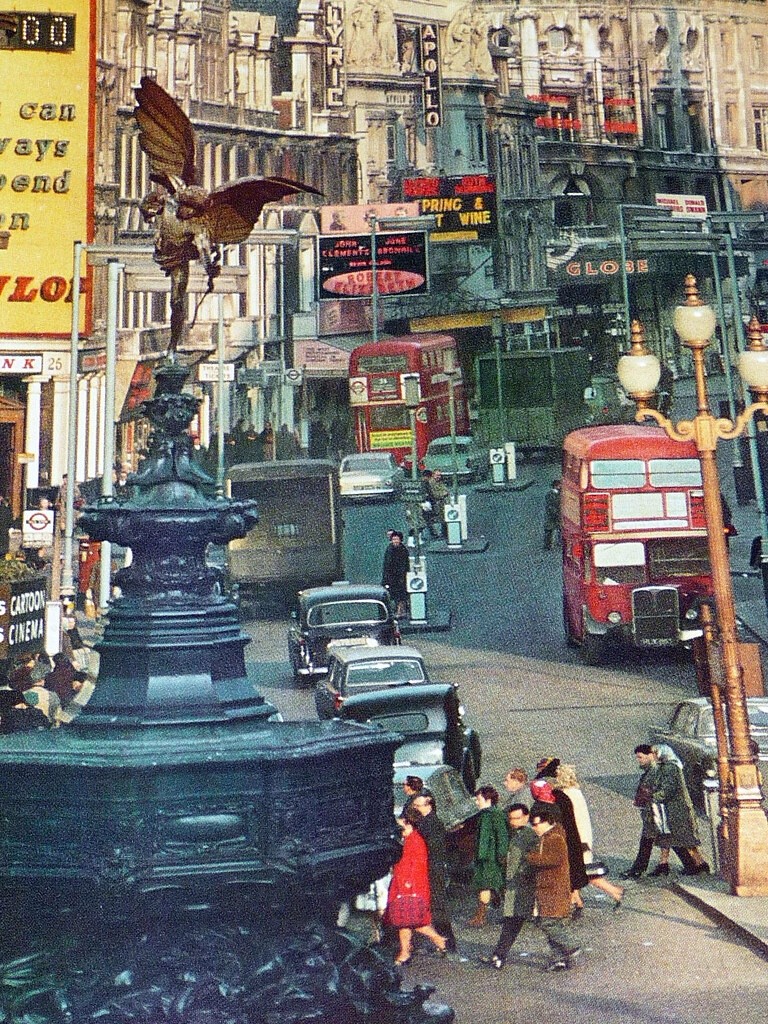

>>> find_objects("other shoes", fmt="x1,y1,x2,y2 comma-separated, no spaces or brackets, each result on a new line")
681,862,709,876
572,899,585,921
569,946,582,961
436,939,456,960
478,953,505,970
621,870,641,882
394,955,413,967
613,888,626,911
546,960,567,973
647,864,670,877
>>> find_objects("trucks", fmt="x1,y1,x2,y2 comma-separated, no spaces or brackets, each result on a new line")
473,347,620,462
224,459,345,604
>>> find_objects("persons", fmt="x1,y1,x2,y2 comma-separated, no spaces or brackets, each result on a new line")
113,462,132,495
39,499,50,509
382,530,411,617
0,489,14,551
618,745,711,878
0,615,85,731
465,758,626,970
57,474,81,532
720,493,732,550
141,184,220,351
75,548,122,620
408,469,449,549
0,549,51,580
192,416,346,477
336,776,457,966
544,480,562,549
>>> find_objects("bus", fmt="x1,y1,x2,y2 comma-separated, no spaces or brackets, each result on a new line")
349,334,472,474
561,426,729,668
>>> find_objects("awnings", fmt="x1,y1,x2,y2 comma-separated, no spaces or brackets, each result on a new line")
293,332,394,378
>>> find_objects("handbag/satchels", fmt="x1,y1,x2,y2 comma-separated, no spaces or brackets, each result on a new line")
587,862,608,878
390,887,427,928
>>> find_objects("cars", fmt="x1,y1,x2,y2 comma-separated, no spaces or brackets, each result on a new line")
418,435,482,485
314,646,431,722
285,581,401,689
389,763,478,834
646,694,766,808
340,451,403,505
342,683,482,794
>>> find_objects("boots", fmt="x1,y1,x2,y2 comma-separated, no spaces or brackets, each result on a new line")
469,900,489,926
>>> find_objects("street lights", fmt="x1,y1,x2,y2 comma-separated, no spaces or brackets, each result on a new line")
617,275,767,898
